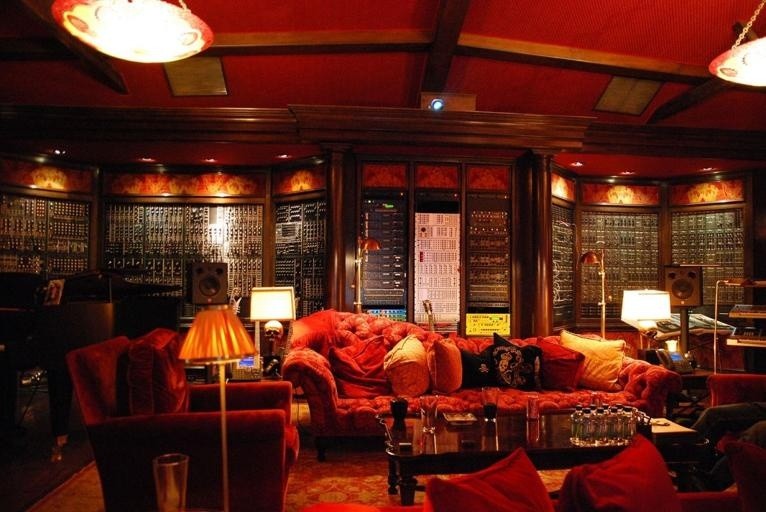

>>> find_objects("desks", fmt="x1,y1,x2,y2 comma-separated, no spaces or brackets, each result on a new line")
374,413,696,506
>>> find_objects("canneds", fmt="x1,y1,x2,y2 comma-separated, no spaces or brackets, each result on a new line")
527,397,539,419
527,419,539,442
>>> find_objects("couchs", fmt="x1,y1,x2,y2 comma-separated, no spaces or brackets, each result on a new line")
285,311,681,461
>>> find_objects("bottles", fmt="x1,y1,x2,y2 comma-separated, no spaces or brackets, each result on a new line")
570,404,636,447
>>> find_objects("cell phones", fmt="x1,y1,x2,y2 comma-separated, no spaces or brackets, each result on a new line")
460,438,474,446
398,440,413,449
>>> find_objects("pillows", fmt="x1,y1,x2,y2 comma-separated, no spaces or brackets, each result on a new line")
559,329,626,391
421,446,556,512
383,334,429,397
490,332,542,392
128,328,190,414
556,431,683,512
425,338,462,395
329,334,390,400
461,345,493,388
534,336,585,393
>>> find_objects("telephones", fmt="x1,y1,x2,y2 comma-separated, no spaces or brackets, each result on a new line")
655,348,694,374
226,357,264,382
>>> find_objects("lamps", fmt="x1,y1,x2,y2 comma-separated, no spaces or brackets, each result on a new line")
581,241,605,339
620,290,671,350
709,1,766,86
177,309,258,512
354,236,380,313
249,287,297,356
49,0,214,63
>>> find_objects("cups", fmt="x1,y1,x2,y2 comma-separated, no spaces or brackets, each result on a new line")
152,453,190,512
419,396,438,433
527,394,539,419
482,387,500,421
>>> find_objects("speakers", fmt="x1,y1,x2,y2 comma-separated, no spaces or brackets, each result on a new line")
663,264,703,309
186,262,228,306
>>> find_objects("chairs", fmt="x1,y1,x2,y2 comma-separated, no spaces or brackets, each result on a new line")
708,374,766,452
67,336,300,512
